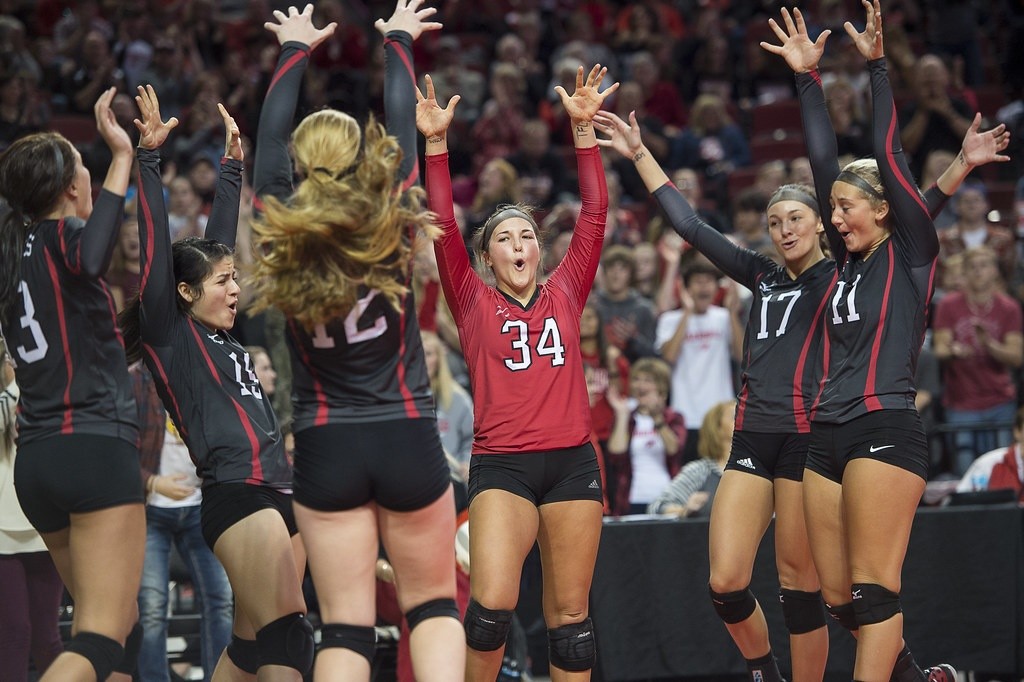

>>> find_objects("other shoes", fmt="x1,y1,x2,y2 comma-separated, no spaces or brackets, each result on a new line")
923,664,958,682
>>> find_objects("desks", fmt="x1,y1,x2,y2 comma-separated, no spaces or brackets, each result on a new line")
515,504,1024,682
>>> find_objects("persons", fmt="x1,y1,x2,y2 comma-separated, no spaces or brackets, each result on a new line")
759,0,963,682
240,0,467,682
414,65,619,682
0,87,146,682
0,0,1024,682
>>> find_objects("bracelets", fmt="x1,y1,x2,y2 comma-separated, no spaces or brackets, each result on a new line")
150,475,157,493
